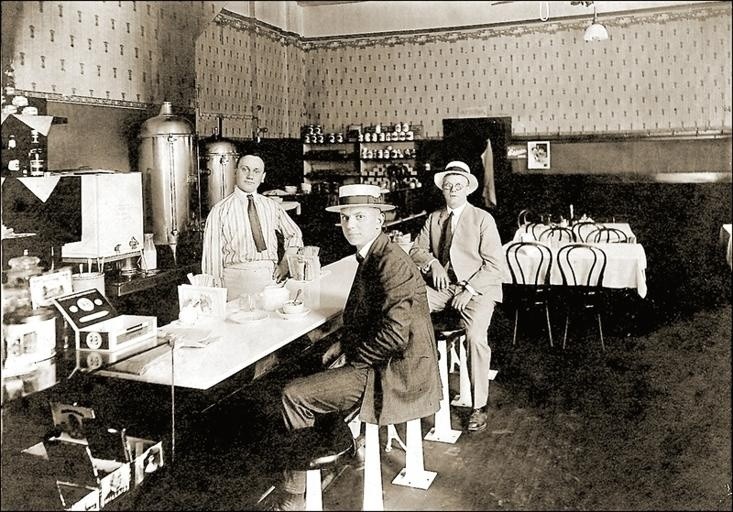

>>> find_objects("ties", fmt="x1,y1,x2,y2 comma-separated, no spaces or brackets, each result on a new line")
245,195,269,252
438,211,455,267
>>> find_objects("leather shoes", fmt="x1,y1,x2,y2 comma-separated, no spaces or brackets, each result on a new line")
310,438,357,468
468,407,488,430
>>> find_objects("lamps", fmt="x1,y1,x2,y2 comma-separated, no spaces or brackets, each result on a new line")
582,1,611,42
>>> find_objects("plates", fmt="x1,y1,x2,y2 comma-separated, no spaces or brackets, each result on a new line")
275,307,312,319
226,310,272,325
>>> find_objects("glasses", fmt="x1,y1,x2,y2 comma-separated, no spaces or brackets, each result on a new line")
442,183,465,192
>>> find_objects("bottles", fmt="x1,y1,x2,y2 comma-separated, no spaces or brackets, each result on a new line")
142,232,158,273
292,256,314,281
27,129,46,178
5,134,23,177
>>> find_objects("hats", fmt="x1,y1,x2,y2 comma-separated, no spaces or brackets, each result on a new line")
324,184,396,212
434,161,479,196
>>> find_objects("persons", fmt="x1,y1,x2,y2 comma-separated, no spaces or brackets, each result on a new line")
201,153,303,301
408,160,506,433
266,185,443,512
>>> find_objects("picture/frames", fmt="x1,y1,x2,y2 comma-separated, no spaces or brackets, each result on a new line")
525,139,552,172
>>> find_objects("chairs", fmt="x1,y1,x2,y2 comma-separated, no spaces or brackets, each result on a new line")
500,208,646,354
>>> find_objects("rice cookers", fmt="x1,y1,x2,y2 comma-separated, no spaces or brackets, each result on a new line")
1,302,60,370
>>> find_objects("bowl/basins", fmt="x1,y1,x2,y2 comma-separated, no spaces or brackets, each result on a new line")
284,186,298,195
300,182,312,193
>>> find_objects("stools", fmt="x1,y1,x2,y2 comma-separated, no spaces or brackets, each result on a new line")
423,317,470,448
262,425,355,512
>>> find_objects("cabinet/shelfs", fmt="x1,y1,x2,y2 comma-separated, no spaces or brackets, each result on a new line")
299,122,422,193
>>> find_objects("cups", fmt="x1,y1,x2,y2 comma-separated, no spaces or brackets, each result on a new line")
238,293,266,312
282,300,305,314
